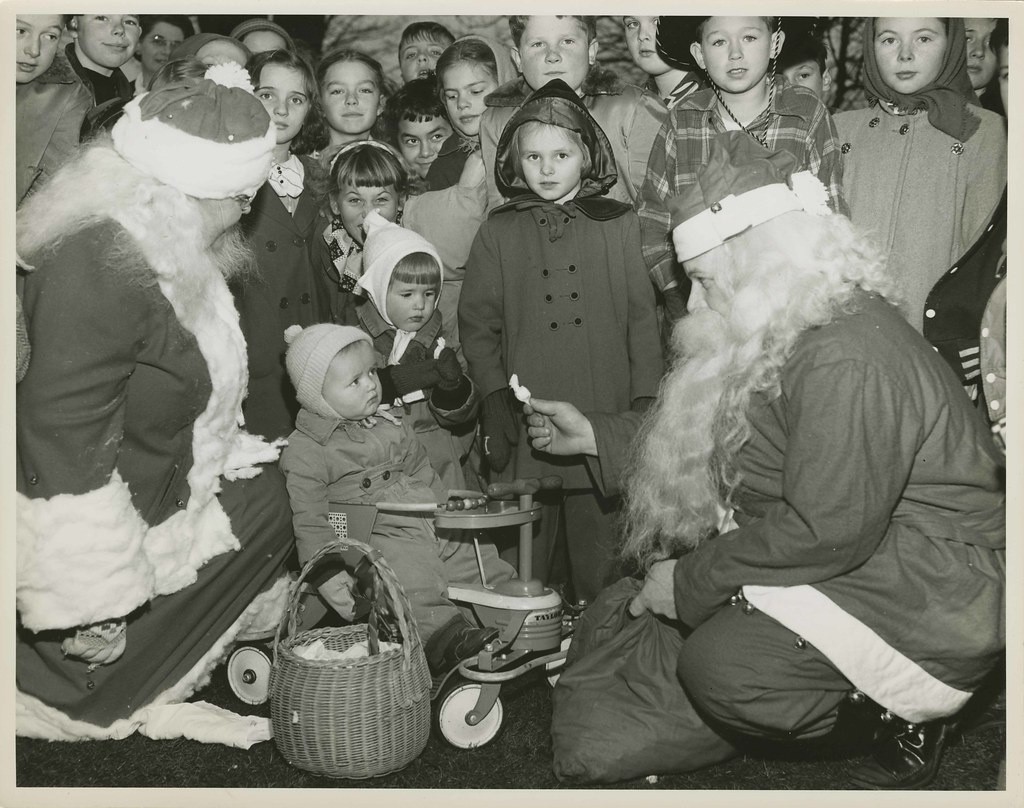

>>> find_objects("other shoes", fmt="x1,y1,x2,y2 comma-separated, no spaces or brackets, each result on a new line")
453,625,503,665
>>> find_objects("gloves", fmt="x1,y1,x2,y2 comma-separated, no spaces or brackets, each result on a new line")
481,391,524,470
389,359,442,396
60,616,130,666
434,346,470,394
321,566,364,625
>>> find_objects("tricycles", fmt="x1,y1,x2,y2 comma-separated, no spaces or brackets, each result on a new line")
225,474,584,751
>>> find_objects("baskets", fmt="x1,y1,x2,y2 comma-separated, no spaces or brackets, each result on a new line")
278,537,433,781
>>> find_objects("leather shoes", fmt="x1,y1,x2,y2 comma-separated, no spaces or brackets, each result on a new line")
852,708,961,790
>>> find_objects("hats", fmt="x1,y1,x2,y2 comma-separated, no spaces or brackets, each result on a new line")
667,129,831,266
112,77,284,199
283,322,378,422
654,12,814,71
359,211,444,328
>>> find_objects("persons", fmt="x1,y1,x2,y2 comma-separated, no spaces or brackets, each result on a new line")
459,80,662,600
524,132,1006,791
16,15,1010,450
284,323,518,679
351,210,480,492
16,62,303,740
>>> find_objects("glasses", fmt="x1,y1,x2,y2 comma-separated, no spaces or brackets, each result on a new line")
150,34,186,55
236,190,257,211
